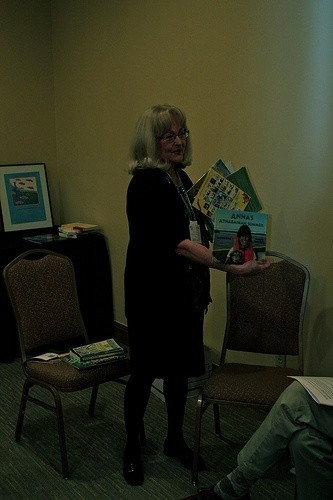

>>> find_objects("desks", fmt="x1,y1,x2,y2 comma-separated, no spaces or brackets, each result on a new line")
0,226,113,365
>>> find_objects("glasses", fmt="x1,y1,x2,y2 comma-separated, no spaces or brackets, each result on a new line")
156,129,189,144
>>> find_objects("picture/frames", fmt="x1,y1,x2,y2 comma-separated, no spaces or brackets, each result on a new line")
0,163,55,237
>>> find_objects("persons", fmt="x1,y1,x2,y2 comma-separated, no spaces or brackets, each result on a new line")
123,105,271,487
287,376,333,406
226,225,257,264
179,379,333,500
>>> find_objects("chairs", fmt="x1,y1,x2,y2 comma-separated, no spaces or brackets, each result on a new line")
192,251,311,487
3,249,132,481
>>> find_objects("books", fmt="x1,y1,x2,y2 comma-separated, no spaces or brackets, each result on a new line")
60,338,128,371
186,159,265,243
23,234,69,244
212,207,269,264
58,222,101,239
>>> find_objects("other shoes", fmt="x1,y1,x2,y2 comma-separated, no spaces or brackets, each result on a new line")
123,445,143,486
181,483,251,500
163,439,207,471
